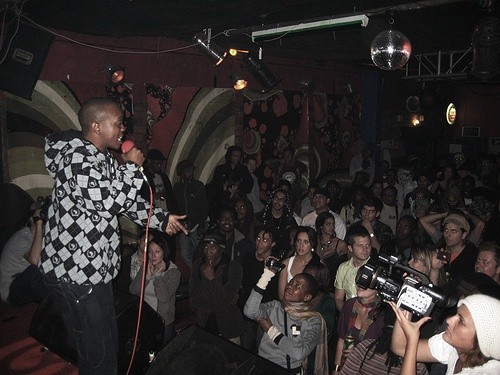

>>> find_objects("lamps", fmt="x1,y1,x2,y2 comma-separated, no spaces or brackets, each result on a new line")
370,12,412,70
244,55,283,94
229,71,248,91
252,15,369,41
445,102,457,125
106,65,127,85
195,35,227,67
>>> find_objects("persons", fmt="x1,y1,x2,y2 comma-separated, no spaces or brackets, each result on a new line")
239,227,285,354
190,230,243,348
0,217,58,309
37,98,191,375
243,255,329,375
426,273,500,374
278,226,319,302
328,286,393,374
383,294,500,375
171,160,209,289
117,235,183,374
303,261,336,374
205,145,500,286
113,230,155,296
333,224,372,311
342,307,430,375
139,149,178,263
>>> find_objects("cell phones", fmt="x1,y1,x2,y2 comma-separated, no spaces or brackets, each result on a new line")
437,251,451,264
266,258,286,274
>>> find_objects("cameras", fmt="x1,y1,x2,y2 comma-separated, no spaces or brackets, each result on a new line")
354,251,464,325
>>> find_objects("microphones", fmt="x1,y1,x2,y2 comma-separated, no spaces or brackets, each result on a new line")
121,140,144,173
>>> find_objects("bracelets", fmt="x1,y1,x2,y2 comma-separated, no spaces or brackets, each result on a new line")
332,363,341,371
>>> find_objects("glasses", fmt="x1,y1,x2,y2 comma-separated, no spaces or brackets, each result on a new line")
295,239,311,244
255,236,269,243
362,208,376,214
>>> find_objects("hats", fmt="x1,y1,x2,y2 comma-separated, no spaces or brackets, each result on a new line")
176,159,197,176
203,230,227,246
442,213,470,236
456,294,500,360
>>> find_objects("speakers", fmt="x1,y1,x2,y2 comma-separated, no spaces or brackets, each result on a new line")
0,18,56,102
